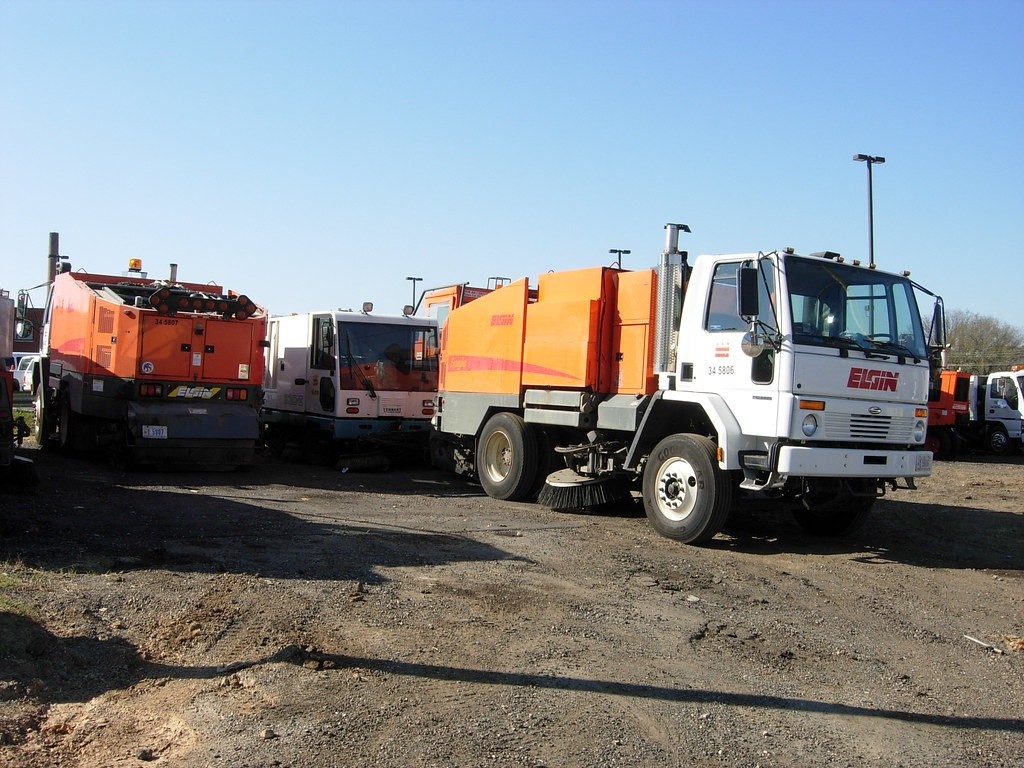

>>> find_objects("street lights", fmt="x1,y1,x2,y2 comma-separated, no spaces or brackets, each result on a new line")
406,276,422,311
854,153,887,337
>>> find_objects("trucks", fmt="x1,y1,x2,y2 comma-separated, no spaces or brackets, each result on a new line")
423,221,948,546
926,364,1024,455
261,302,439,447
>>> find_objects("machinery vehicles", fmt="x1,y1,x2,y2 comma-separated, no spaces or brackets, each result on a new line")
405,277,512,361
9,232,273,453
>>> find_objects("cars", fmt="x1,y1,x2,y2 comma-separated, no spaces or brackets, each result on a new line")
12,352,41,393
